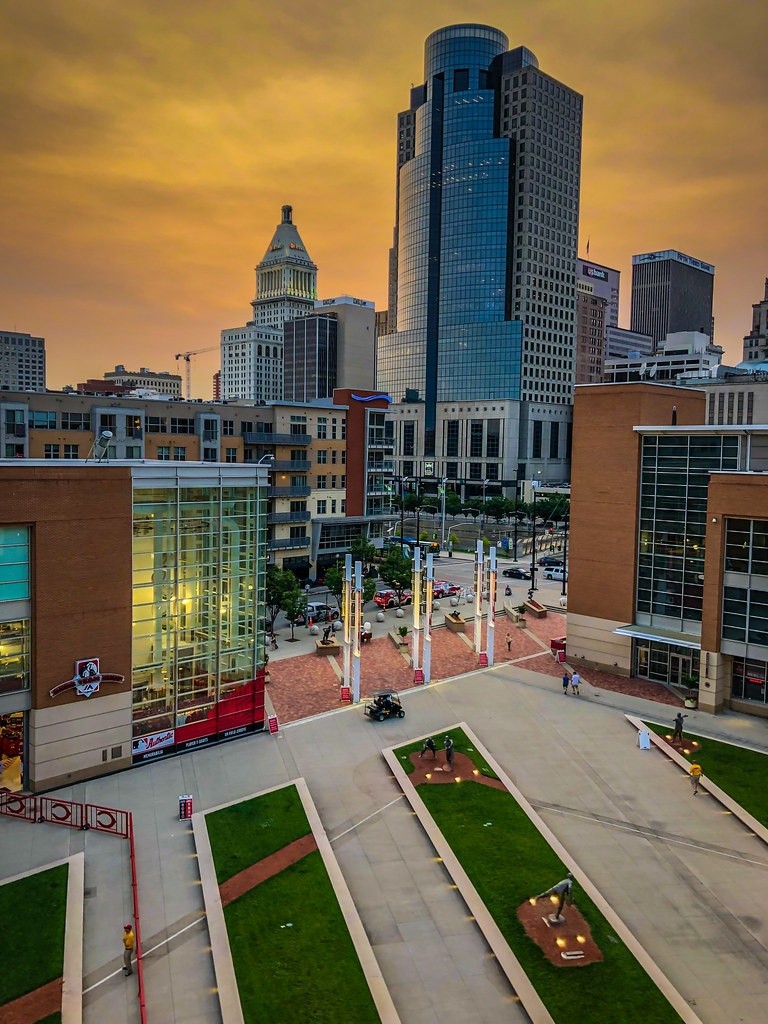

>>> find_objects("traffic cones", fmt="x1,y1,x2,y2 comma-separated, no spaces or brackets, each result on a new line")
308,616,313,626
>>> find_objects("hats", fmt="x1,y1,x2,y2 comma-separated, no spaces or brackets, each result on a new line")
124,925,132,930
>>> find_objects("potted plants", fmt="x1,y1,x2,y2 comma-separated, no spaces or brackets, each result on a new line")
518,606,526,627
399,626,408,652
685,674,698,708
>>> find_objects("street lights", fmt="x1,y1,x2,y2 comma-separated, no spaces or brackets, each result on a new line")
304,584,310,628
400,475,409,555
441,477,449,551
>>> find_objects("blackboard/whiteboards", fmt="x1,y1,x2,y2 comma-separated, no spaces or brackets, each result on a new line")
636,732,650,750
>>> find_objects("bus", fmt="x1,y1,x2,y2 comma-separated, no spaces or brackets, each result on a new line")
407,541,441,562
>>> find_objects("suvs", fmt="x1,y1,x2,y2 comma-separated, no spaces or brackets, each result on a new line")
297,601,340,625
372,588,412,609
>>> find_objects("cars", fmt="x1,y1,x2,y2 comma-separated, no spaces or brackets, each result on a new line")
536,556,564,567
542,566,568,582
502,566,532,580
433,579,461,599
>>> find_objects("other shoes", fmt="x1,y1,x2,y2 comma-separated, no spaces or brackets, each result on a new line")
573,693,575,694
693,791,698,795
564,692,567,694
577,691,579,694
123,967,128,970
125,972,132,976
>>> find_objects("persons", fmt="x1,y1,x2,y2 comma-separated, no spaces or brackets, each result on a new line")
689,760,702,795
376,694,393,710
0,720,17,735
535,873,574,920
670,712,684,746
528,589,534,603
451,610,460,618
505,632,512,652
563,673,569,695
504,585,512,606
420,736,436,758
142,689,173,712
322,626,331,645
331,620,338,637
270,634,276,651
443,735,454,771
122,925,135,977
570,672,582,695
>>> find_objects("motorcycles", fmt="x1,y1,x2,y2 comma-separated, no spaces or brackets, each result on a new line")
363,689,406,722
